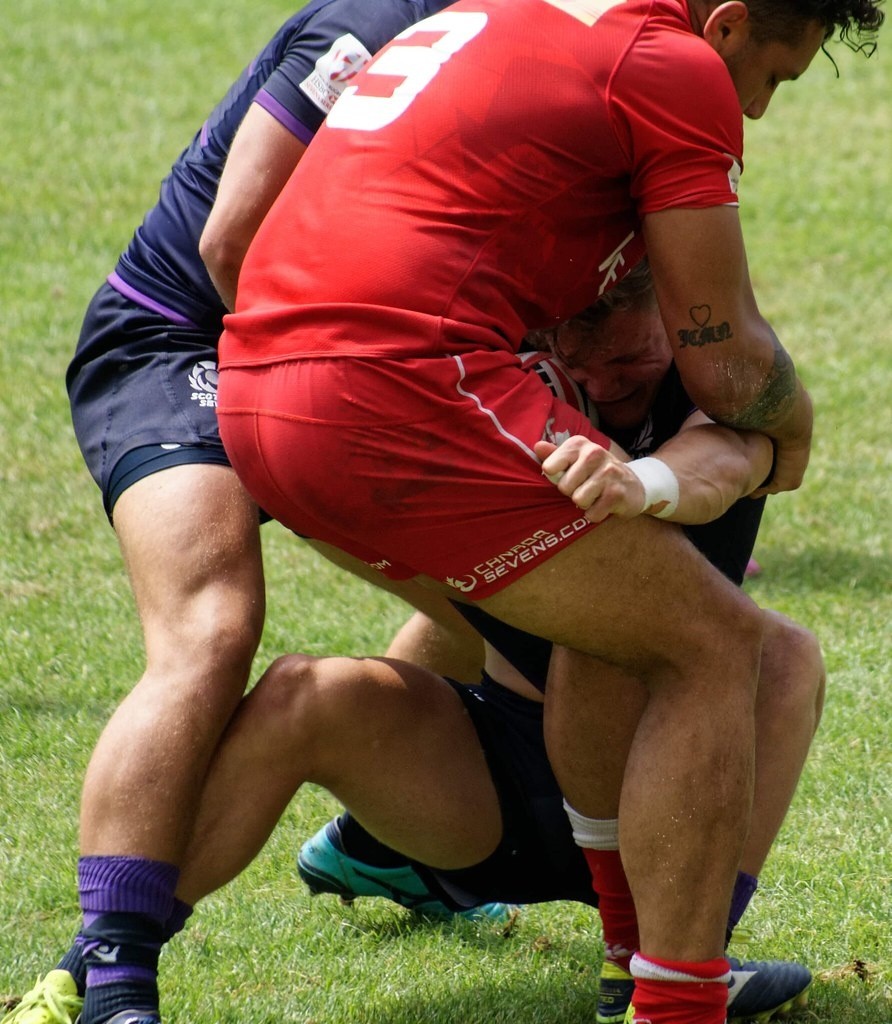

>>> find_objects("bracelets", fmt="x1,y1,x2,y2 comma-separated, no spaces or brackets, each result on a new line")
625,456,680,519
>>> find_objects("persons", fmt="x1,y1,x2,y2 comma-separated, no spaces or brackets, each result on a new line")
0,0,884,1024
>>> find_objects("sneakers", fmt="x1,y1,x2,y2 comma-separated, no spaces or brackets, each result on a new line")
595,953,814,1024
0,969,84,1024
297,816,510,934
